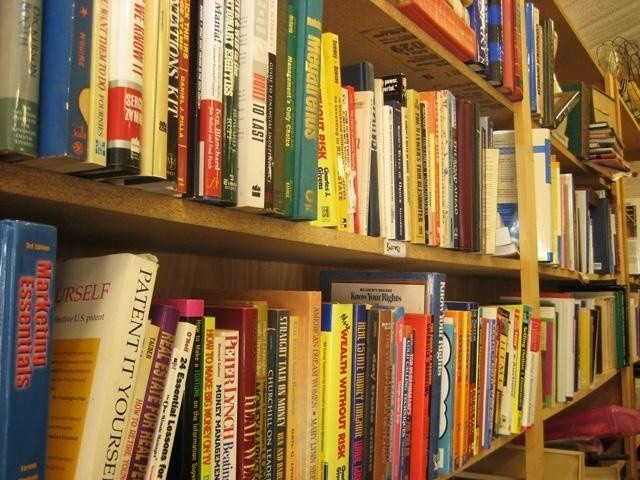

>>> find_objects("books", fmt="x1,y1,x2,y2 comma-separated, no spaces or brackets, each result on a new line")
2,0,639,479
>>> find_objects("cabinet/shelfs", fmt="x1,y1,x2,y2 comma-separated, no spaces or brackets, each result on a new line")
0,0,640,479
